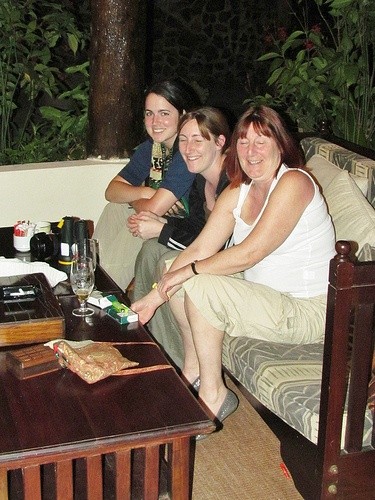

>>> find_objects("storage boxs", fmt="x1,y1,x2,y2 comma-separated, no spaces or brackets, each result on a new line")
6,344,62,380
0,273,66,347
86,291,139,324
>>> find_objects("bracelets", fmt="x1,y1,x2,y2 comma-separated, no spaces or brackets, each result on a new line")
151,282,158,290
191,259,200,275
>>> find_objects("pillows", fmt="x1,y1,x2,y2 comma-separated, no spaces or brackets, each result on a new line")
306,153,375,256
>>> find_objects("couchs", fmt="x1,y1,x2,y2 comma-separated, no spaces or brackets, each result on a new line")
222,137,375,500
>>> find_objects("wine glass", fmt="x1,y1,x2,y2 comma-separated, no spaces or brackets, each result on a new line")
70,257,96,317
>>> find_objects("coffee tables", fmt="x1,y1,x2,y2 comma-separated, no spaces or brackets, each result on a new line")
0,222,224,500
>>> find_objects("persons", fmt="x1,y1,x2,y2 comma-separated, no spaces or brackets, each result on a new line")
130,106,246,374
128,103,339,441
88,76,203,294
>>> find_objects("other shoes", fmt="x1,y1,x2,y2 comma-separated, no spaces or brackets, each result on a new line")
190,387,238,440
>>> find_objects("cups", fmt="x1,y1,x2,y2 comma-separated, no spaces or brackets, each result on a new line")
13,235,32,252
73,239,97,272
34,222,51,234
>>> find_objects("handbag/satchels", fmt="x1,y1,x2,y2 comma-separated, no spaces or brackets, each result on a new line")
53,341,140,385
